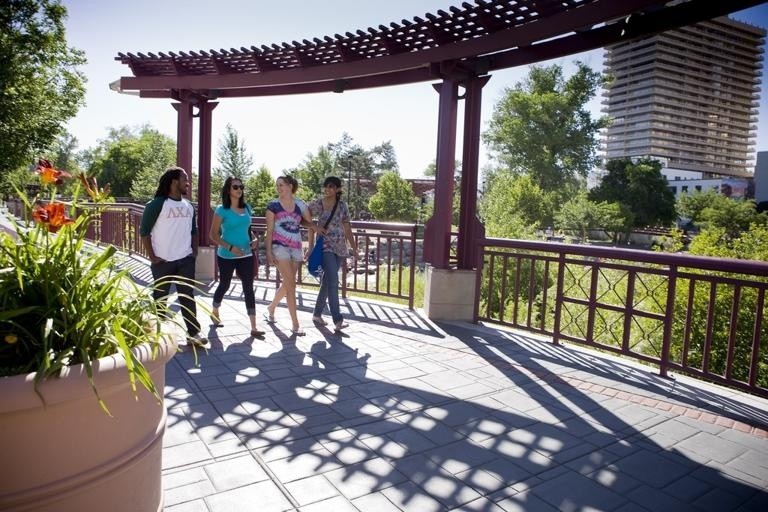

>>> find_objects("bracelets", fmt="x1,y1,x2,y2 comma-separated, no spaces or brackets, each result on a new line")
229,245,233,251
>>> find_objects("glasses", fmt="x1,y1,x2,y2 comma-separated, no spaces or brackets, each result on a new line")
231,185,245,190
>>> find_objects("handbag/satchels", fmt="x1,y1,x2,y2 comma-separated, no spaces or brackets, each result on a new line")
307,236,324,278
252,252,259,278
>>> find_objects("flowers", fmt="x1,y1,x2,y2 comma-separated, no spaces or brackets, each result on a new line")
0,154,222,416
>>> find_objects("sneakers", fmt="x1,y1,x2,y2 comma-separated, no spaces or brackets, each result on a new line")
311,315,328,326
333,321,350,331
186,336,208,347
210,313,224,327
250,328,266,336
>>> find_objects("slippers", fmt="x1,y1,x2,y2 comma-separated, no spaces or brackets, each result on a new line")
263,311,277,323
290,326,306,336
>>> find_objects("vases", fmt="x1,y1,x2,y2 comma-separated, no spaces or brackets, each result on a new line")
0,310,180,511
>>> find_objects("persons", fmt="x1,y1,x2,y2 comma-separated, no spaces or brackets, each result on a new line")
301,177,357,331
266,175,315,335
209,176,265,336
140,168,208,347
